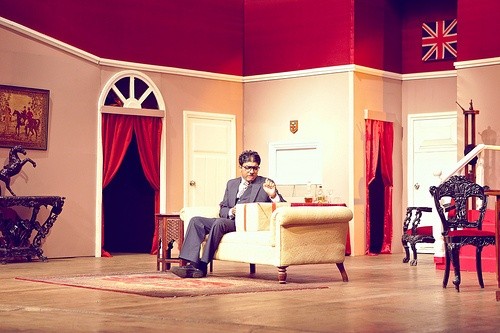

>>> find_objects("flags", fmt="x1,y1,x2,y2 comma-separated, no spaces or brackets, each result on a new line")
421,19,458,61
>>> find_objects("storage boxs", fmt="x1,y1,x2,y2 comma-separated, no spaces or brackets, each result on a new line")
234,202,292,233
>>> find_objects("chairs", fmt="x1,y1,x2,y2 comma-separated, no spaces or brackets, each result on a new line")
429,175,496,291
402,203,450,267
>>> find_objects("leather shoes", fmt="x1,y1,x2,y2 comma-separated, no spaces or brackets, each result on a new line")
170,265,206,278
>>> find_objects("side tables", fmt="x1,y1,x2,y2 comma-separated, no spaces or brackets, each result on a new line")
155,214,186,271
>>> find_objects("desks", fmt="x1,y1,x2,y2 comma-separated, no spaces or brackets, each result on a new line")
0,195,66,264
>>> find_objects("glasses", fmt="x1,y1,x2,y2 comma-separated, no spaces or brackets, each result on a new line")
242,165,261,170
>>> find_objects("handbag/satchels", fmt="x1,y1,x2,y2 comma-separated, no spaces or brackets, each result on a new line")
234,201,272,231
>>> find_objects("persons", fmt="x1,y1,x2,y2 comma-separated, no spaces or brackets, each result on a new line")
170,150,287,278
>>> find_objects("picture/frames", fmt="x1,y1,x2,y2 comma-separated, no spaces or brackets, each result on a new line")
0,85,50,150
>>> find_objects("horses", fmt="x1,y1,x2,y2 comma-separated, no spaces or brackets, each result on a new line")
0,145,36,196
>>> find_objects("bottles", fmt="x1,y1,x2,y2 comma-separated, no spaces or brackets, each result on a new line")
317,186,325,203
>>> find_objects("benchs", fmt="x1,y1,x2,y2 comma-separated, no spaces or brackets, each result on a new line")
180,206,354,283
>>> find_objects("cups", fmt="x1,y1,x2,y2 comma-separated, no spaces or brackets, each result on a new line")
304,193,313,203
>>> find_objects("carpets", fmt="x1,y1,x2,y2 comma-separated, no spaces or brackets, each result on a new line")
14,274,329,297
